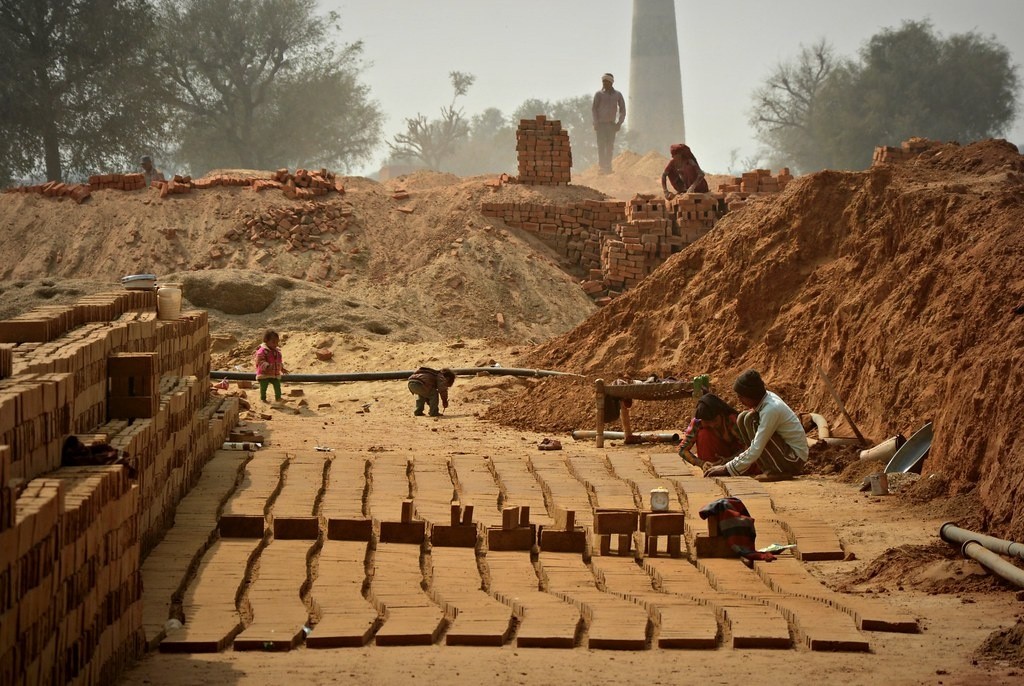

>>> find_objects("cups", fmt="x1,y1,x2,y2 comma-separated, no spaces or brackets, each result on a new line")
651,489,669,509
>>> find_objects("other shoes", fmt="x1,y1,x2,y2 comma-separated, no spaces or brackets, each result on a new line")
754,470,794,482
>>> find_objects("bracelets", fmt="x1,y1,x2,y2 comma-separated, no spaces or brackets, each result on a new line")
663,189,668,192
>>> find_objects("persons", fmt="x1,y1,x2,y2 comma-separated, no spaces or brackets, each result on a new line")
592,73,626,177
254,329,289,404
140,154,165,187
704,370,810,480
661,144,709,201
406,367,456,417
677,394,762,476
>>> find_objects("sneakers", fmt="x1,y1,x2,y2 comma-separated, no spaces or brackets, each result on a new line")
539,437,562,450
